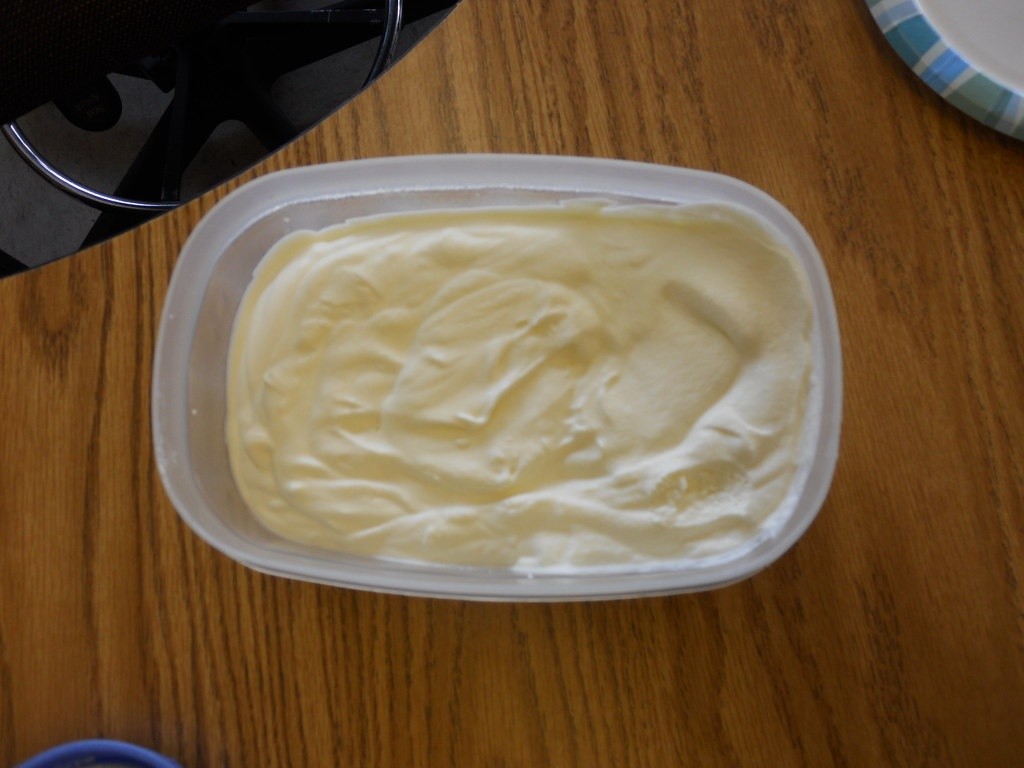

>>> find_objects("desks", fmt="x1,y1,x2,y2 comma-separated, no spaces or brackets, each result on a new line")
0,0,1024,768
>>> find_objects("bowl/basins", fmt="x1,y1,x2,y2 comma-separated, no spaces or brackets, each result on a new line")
14,738,181,768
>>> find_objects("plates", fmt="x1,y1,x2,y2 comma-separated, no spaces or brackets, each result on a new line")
865,0,1024,144
